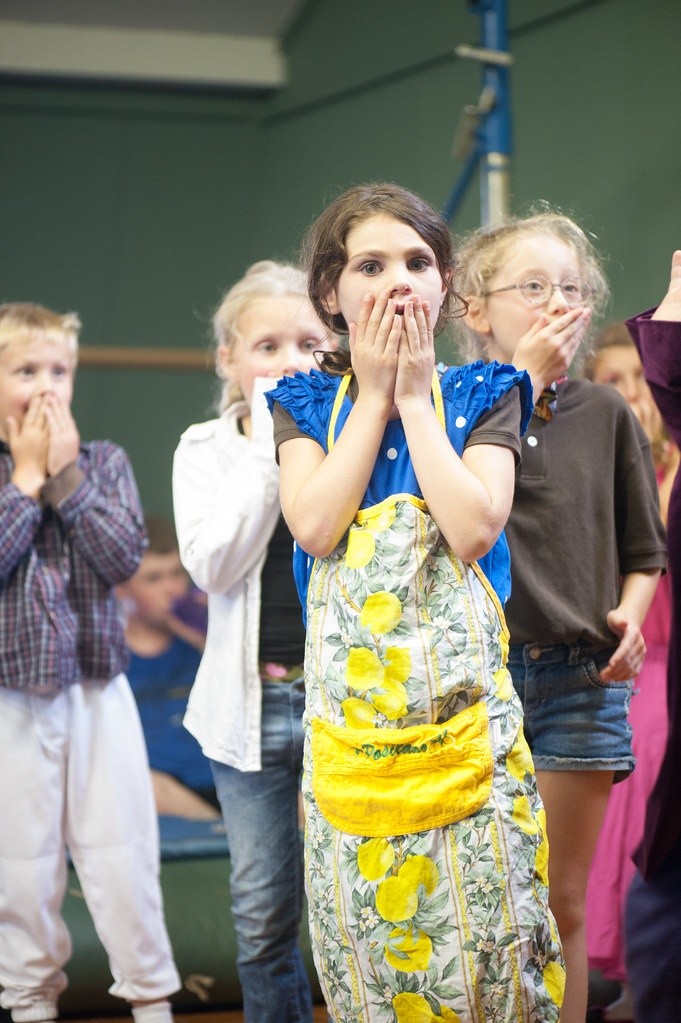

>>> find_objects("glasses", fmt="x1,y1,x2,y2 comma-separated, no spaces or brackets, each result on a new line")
483,272,596,308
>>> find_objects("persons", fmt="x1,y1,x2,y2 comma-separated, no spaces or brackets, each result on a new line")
0,297,180,1023
624,231,681,1022
453,209,668,1023
103,507,220,817
171,261,391,1023
573,325,679,1023
263,180,565,1022
